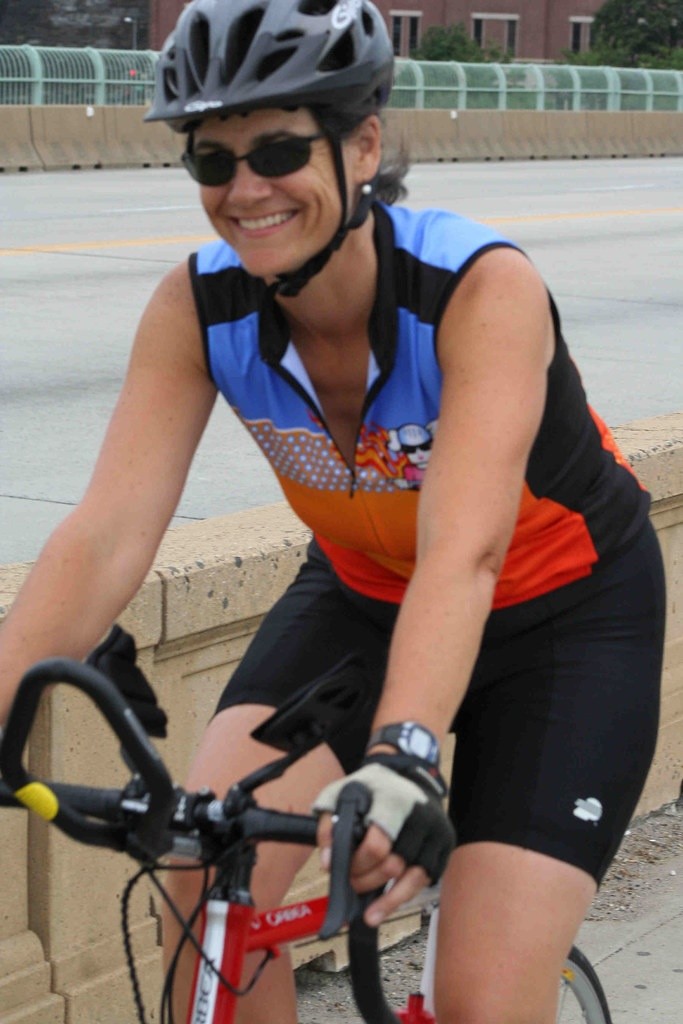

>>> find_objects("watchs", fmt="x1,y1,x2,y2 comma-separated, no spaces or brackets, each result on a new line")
367,720,440,766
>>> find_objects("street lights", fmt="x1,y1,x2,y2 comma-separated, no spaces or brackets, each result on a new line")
123,17,137,50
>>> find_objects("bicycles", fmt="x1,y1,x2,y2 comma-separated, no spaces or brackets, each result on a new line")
1,657,617,1023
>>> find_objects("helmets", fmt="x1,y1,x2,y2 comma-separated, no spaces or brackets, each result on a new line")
143,0,392,121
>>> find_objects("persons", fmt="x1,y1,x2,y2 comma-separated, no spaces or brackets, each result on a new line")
0,0,667,1024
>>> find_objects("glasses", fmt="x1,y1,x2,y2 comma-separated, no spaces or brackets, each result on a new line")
181,132,326,185
